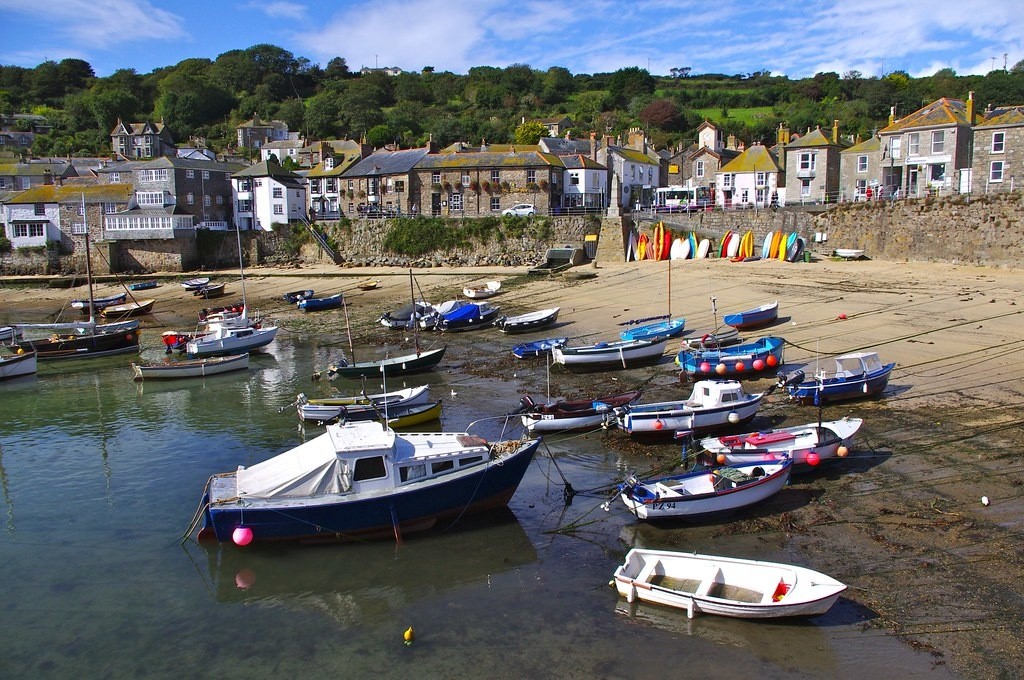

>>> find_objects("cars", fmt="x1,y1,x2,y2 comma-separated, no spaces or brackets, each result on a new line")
502,204,538,218
360,205,396,218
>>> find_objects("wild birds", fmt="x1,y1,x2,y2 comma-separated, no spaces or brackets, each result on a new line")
403,626,416,646
982,495,993,507
451,389,458,396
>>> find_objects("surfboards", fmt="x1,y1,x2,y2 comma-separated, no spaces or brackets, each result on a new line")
626,220,804,262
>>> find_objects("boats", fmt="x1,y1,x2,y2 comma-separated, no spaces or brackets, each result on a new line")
521,390,643,434
357,281,378,291
192,283,225,300
681,327,738,347
700,417,865,466
198,420,541,546
551,342,666,374
297,294,343,314
70,292,126,316
160,304,278,360
675,337,785,378
180,277,209,291
617,458,794,522
626,222,807,263
131,353,250,383
609,549,847,619
283,290,315,304
619,317,685,343
297,383,441,429
723,301,778,329
379,300,500,333
1,190,141,380
129,281,157,291
329,268,447,378
613,379,765,438
512,336,570,359
836,249,865,260
496,307,561,333
99,299,155,319
462,280,502,299
784,351,896,405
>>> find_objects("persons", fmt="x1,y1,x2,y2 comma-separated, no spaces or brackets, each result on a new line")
865,185,872,201
309,207,316,221
879,184,884,200
357,204,363,216
854,186,859,202
769,191,780,208
381,204,401,218
411,204,417,218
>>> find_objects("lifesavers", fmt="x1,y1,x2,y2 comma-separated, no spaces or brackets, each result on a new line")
701,334,716,352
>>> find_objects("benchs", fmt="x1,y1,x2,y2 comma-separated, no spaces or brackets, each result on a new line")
760,574,783,605
695,565,720,597
635,559,659,583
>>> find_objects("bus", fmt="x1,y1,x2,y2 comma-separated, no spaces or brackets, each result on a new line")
650,185,711,213
